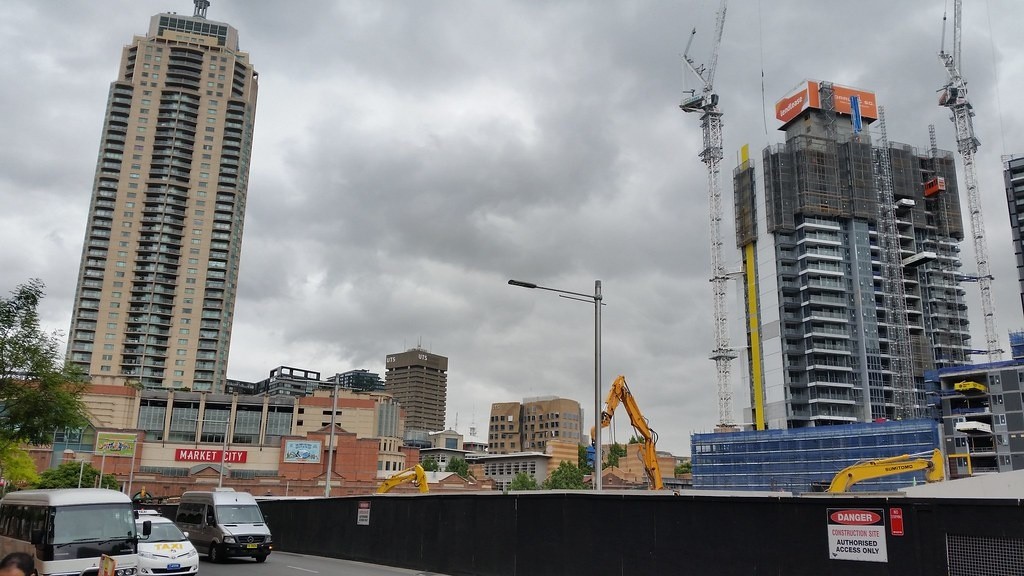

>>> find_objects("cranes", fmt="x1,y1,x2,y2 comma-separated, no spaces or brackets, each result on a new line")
935,1,1005,364
677,1,740,433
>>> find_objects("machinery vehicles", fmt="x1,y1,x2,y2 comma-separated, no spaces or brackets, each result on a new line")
585,375,665,490
827,448,946,492
377,463,430,494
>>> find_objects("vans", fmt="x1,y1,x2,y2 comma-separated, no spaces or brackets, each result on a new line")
174,489,274,563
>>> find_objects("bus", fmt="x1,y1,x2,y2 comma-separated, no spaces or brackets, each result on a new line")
1,487,135,575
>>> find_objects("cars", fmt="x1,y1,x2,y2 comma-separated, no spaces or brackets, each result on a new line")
134,508,199,575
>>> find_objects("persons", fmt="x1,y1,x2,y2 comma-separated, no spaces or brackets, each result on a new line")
0,551,37,576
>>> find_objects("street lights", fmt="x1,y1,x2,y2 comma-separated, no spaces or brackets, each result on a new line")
275,373,363,498
180,417,236,488
73,446,118,488
507,279,606,490
98,434,146,497
58,456,96,488
